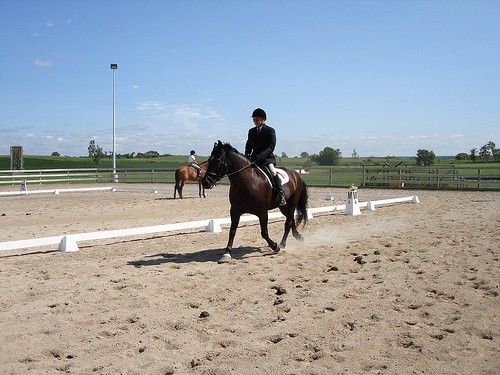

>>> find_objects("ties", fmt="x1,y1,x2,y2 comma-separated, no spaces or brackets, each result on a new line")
257,127,260,133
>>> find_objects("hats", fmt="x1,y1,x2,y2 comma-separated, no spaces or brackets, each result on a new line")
190,151,195,155
252,108,266,120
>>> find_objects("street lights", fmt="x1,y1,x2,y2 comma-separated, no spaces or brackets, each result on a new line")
111,63,118,181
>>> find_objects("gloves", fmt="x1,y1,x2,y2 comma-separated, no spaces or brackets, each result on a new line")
245,150,251,158
255,155,263,161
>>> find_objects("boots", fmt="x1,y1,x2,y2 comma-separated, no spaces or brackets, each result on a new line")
274,175,287,205
197,168,200,180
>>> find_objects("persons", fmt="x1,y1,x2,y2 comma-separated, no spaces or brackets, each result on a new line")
245,108,286,207
189,150,202,180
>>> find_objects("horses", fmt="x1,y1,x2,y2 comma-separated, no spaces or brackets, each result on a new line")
202,139,309,264
173,160,206,199
